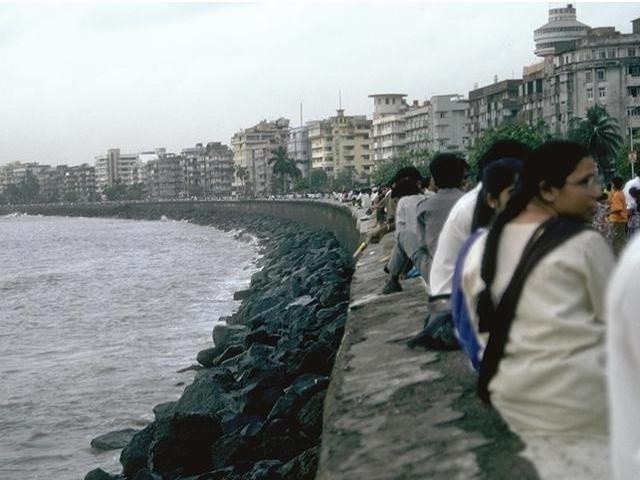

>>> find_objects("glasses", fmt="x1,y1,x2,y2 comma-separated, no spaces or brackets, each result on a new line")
564,175,604,191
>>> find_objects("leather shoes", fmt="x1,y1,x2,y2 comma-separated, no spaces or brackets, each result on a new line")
379,281,402,294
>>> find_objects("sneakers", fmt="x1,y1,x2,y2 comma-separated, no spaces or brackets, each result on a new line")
360,215,370,221
